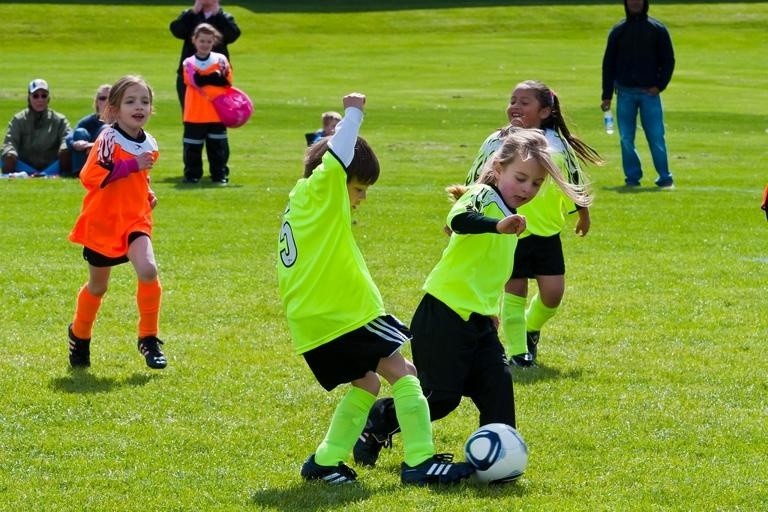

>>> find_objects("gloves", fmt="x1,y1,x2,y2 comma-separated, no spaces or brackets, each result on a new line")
3,153,16,172
61,149,72,171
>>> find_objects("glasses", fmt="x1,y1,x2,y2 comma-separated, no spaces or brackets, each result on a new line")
98,96,107,100
32,94,48,98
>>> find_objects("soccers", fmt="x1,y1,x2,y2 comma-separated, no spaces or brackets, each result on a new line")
465,423,527,483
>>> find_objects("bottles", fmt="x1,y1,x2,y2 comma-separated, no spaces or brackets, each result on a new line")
603,105,614,134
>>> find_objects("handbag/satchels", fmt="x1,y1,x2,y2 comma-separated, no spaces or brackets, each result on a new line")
212,87,253,127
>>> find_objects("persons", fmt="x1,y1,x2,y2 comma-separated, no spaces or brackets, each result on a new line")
178,23,235,187
64,80,113,177
312,107,341,139
66,73,173,372
2,73,73,181
596,3,676,188
342,112,596,476
466,76,609,367
167,1,243,127
277,91,475,486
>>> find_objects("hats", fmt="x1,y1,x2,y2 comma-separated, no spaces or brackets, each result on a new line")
29,79,49,93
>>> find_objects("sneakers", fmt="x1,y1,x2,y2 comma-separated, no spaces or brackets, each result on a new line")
354,398,400,466
510,353,539,370
69,324,90,367
138,335,166,369
302,454,357,486
182,177,200,183
213,177,228,186
527,331,540,360
401,454,475,484
